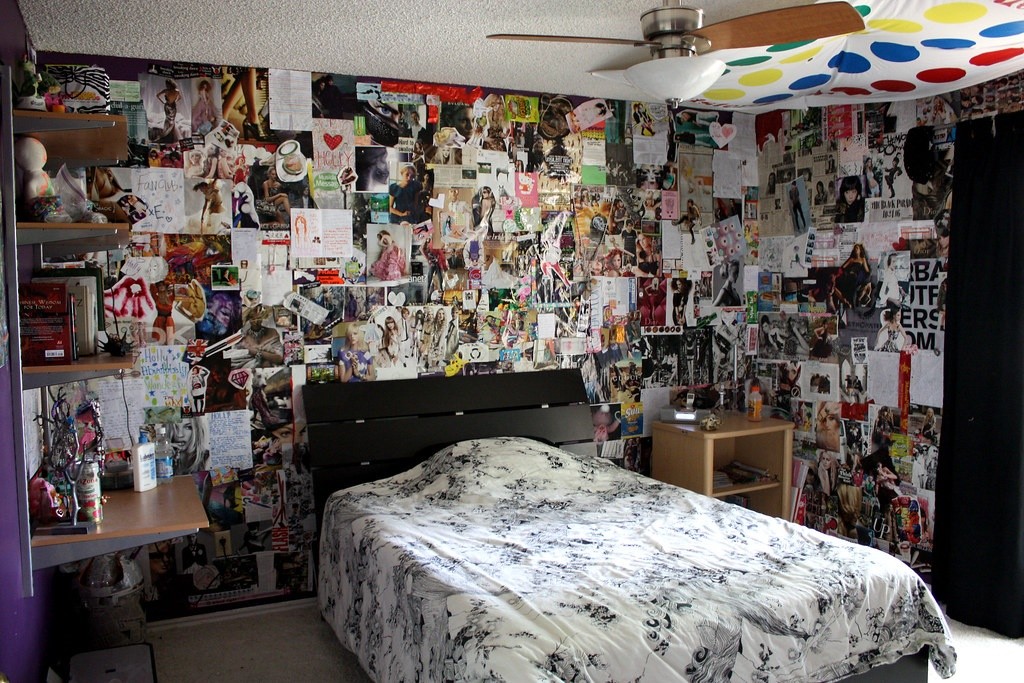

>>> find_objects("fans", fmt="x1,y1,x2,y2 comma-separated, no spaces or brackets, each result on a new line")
485,1,865,59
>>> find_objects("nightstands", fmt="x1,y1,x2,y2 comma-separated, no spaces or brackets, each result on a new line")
651,413,795,521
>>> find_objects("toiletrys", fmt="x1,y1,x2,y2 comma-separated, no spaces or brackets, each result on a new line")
132,430,158,493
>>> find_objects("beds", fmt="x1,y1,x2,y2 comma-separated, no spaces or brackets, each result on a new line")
301,367,959,683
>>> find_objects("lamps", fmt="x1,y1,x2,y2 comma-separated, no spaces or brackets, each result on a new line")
624,57,727,109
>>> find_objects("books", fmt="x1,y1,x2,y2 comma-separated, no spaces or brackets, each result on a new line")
17,268,104,367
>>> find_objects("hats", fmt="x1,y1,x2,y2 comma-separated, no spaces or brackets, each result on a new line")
903,125,936,185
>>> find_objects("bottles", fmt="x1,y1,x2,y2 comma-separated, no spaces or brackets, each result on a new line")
747,386,762,422
154,427,174,486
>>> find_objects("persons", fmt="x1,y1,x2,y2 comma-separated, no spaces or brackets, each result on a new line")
88,65,1024,590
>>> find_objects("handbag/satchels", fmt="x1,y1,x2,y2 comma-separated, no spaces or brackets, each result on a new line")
45,65,111,114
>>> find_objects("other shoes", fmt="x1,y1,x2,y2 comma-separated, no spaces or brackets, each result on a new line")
672,220,677,226
890,193,896,198
691,240,695,244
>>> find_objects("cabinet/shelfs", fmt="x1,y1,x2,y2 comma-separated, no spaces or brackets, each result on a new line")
0,64,211,599
